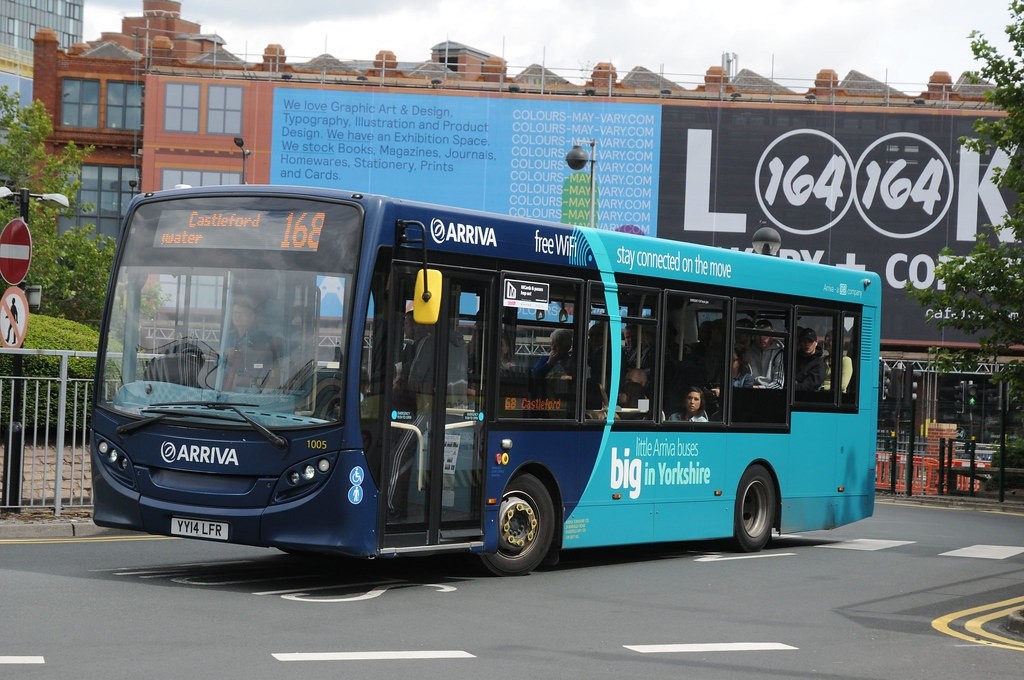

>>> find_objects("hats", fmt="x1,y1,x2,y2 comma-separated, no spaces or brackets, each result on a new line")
799,327,817,341
754,320,773,331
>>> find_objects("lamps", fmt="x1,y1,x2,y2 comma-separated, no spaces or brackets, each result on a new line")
282,74,292,82
585,87,597,96
661,90,671,100
356,76,369,86
730,92,741,103
914,97,926,105
805,94,816,104
432,79,442,89
509,83,520,93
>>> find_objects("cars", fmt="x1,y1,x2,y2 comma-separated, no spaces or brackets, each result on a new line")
931,400,987,441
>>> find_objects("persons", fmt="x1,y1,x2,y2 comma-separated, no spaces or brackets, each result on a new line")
223,296,275,387
387,303,468,524
499,339,519,368
531,329,575,379
360,369,371,416
794,325,852,393
586,316,786,422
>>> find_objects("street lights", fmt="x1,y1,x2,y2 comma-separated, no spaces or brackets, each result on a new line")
231,136,253,183
1,186,70,516
568,139,600,224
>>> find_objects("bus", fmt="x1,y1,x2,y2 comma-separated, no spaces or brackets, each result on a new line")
84,183,881,579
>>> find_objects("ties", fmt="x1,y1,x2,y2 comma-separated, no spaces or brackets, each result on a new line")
224,354,240,387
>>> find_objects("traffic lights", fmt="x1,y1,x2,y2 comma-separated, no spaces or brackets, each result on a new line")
968,397,976,407
968,384,979,396
905,363,921,406
884,369,904,401
953,381,965,414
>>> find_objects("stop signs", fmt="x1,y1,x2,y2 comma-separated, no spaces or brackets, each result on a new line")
1,218,31,286
